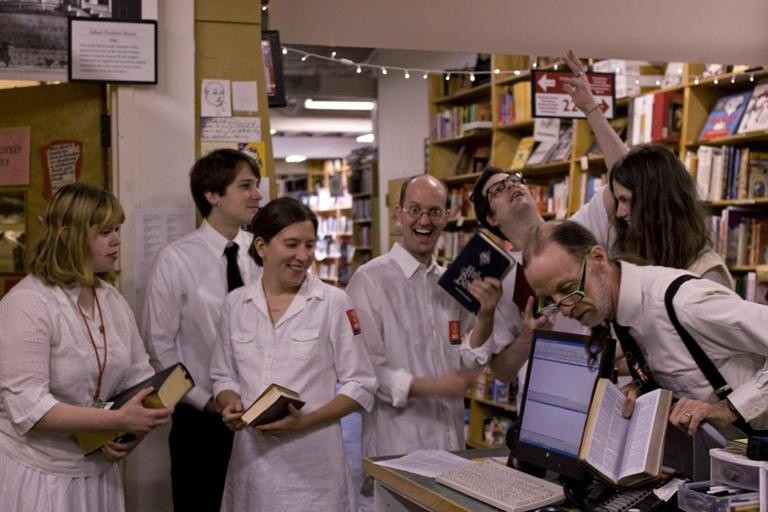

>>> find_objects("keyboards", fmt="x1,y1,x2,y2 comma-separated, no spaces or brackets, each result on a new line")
437,456,566,511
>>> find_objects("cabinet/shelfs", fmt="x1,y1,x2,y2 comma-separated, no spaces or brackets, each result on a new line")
194,1,276,221
308,149,375,286
428,56,768,452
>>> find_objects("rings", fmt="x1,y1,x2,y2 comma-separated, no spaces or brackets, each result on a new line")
684,412,692,417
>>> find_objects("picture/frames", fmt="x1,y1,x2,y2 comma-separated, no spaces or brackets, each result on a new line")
66,15,158,86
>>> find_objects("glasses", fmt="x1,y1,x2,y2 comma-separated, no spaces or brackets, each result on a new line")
484,170,525,203
537,252,589,316
397,204,447,219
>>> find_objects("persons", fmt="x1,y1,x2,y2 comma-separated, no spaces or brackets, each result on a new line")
470,47,629,423
0,182,174,512
142,148,263,510
209,197,380,512
608,143,736,291
345,174,503,498
523,220,768,437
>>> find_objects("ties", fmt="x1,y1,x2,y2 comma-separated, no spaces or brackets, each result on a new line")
222,244,246,293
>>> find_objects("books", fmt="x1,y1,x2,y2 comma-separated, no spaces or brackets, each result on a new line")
297,160,372,285
434,69,627,267
577,377,673,493
632,83,768,305
73,361,195,457
241,384,305,427
437,233,515,312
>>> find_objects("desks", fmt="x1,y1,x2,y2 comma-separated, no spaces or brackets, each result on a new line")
360,440,569,510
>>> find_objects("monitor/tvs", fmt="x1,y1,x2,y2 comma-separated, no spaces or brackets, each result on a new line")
515,325,615,483
